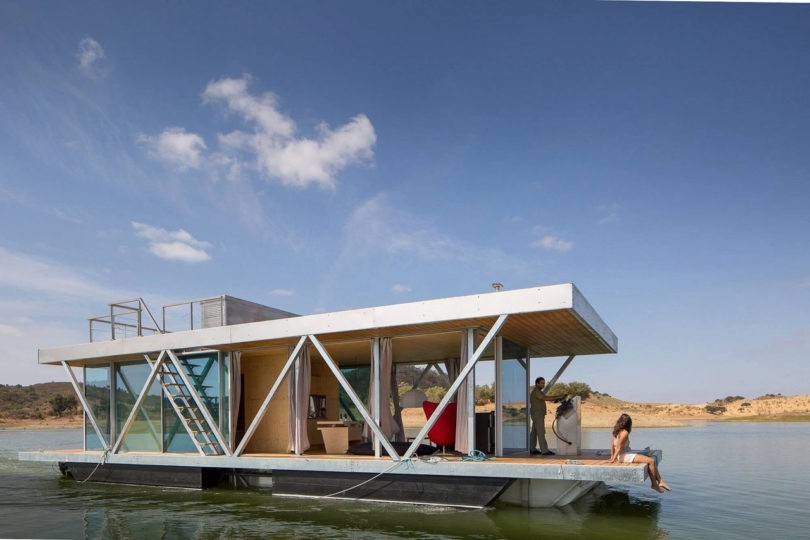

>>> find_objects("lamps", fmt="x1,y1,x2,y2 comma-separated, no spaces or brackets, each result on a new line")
399,375,428,408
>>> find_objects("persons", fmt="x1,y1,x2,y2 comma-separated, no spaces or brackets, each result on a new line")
529,377,566,455
601,414,671,493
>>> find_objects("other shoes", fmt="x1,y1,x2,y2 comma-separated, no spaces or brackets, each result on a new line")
542,450,556,456
530,449,542,455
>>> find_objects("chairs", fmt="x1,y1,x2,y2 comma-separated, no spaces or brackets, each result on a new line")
422,401,457,456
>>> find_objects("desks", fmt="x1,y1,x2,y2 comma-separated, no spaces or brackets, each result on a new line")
316,421,362,454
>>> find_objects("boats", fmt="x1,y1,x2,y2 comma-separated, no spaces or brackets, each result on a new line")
19,281,664,507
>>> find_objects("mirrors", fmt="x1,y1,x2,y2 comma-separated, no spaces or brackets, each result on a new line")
308,395,327,419
